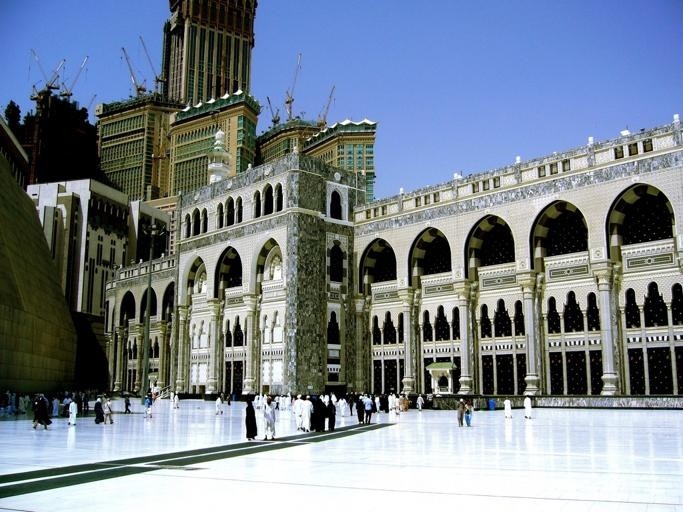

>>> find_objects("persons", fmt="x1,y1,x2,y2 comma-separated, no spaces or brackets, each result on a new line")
226,393,230,406
220,392,224,404
455,398,463,426
470,396,495,412
243,389,424,441
503,396,512,418
214,395,223,416
0,383,181,433
523,395,532,419
463,398,475,426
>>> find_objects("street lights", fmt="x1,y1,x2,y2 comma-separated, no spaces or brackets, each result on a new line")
139,222,167,405
